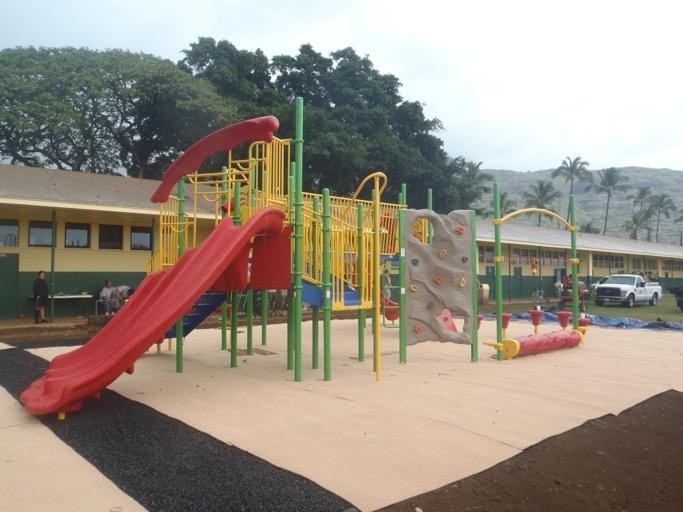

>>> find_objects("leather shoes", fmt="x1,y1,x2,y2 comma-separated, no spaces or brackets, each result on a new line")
34,319,50,325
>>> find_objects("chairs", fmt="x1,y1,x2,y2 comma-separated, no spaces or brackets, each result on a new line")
100,292,102,300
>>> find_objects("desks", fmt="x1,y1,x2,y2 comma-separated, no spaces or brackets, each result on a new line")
95,299,123,315
29,295,93,324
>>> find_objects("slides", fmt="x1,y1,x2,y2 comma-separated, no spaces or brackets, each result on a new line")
19,206,285,420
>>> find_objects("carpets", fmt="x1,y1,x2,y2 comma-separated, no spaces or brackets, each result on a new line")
0,313,683,512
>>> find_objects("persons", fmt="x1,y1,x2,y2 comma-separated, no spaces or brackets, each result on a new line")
97,279,117,316
113,285,136,311
31,270,50,324
380,269,392,299
562,273,572,294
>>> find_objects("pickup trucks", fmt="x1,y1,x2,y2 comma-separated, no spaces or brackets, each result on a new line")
592,273,662,308
